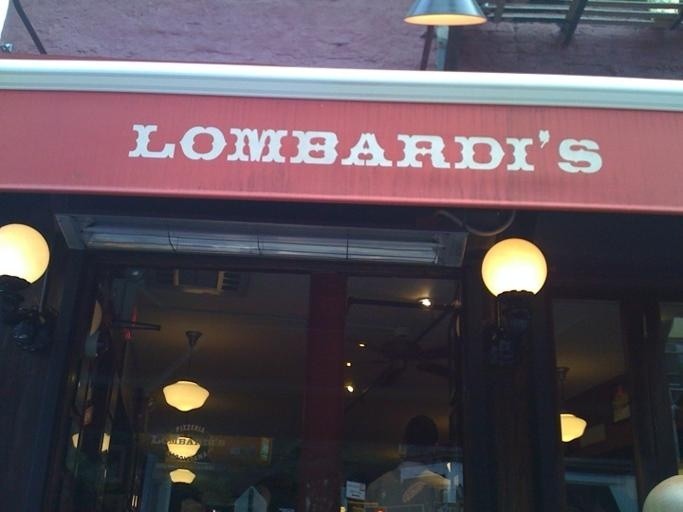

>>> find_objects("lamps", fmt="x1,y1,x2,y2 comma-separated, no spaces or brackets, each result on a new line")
476,236,548,338
402,1,488,27
556,366,587,444
162,330,209,458
0,221,49,323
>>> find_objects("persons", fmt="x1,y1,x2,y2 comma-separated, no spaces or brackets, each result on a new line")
363,415,459,512
